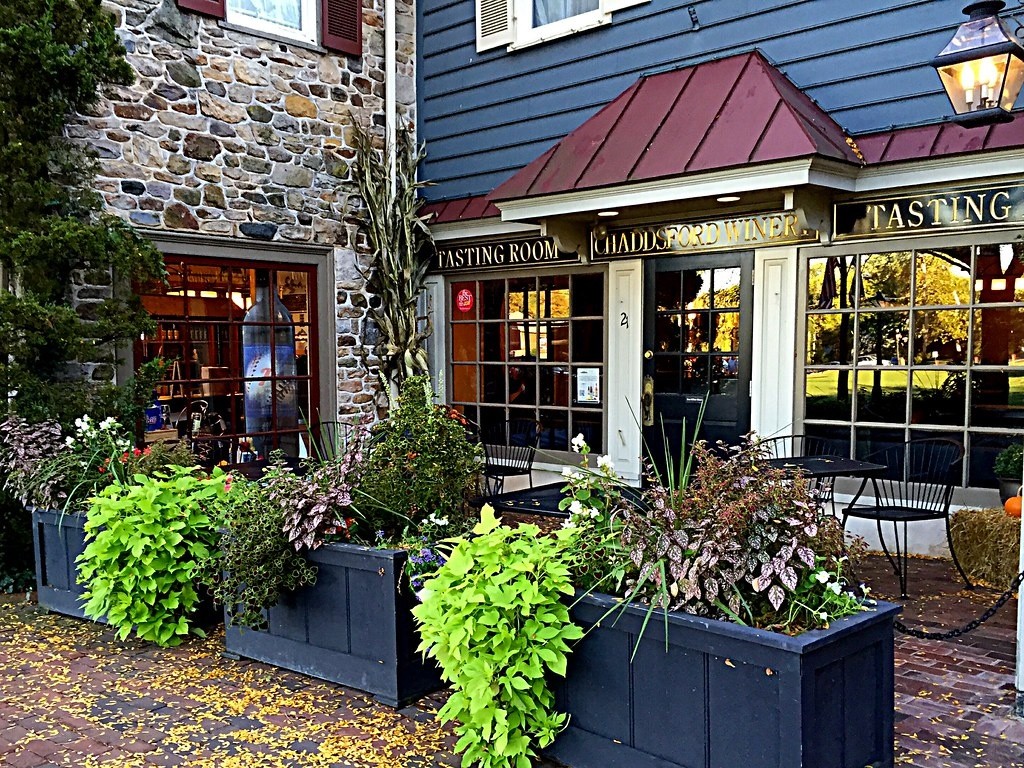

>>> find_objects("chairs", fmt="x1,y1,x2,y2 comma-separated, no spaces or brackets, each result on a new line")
842,438,971,588
306,420,376,470
474,419,541,496
748,436,836,523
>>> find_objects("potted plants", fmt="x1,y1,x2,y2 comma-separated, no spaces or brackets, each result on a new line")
992,430,1024,507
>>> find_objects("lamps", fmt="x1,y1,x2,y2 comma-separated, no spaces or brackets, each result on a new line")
928,0,1024,130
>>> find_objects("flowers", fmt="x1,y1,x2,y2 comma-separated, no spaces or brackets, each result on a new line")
193,369,487,636
412,387,877,768
0,408,250,649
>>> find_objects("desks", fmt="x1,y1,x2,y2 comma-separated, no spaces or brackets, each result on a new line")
741,456,888,586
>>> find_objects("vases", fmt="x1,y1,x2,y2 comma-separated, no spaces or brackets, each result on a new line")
490,587,904,768
215,529,459,709
24,505,138,633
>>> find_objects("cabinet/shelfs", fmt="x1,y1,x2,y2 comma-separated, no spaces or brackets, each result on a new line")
142,315,239,399
